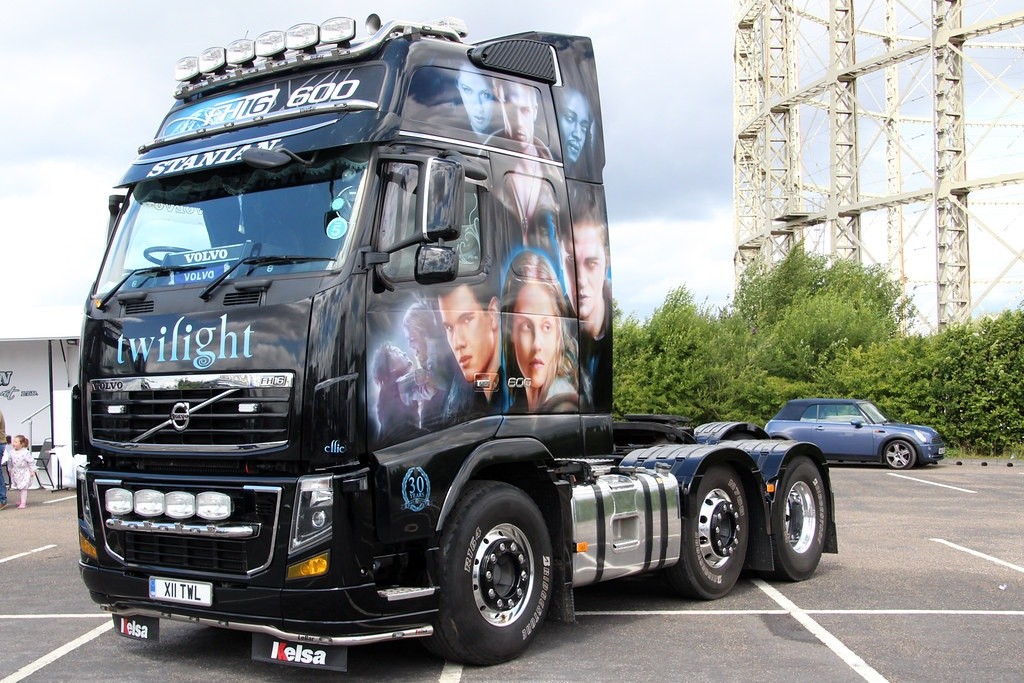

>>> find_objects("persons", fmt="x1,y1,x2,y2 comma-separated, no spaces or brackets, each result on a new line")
0,410,7,507
1,436,13,490
1,435,38,509
475,70,565,295
455,60,501,134
404,303,453,428
550,85,590,178
502,251,599,413
373,335,438,449
436,259,512,427
552,184,613,414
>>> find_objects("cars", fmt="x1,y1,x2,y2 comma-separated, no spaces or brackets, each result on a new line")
763,398,947,470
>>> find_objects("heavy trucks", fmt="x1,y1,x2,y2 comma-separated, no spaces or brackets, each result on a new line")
69,13,841,670
